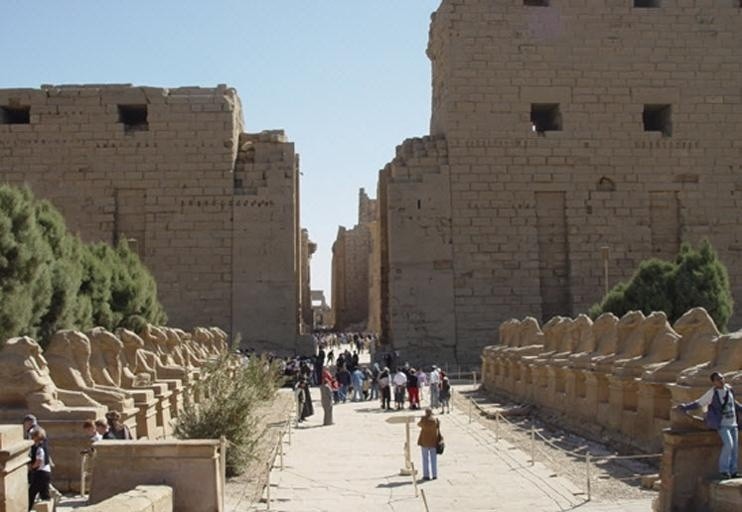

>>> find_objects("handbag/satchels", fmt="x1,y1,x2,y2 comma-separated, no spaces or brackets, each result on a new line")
436,435,444,454
704,406,722,430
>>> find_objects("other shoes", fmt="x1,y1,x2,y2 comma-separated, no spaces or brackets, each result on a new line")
719,470,742,479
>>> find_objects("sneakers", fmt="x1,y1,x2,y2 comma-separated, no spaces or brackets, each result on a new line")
422,476,437,481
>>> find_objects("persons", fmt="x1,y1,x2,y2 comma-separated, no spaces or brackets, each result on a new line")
21,413,64,505
236,344,451,426
675,371,741,478
106,410,136,439
416,405,441,481
27,425,57,512
312,329,381,354
80,419,105,456
94,419,116,440
